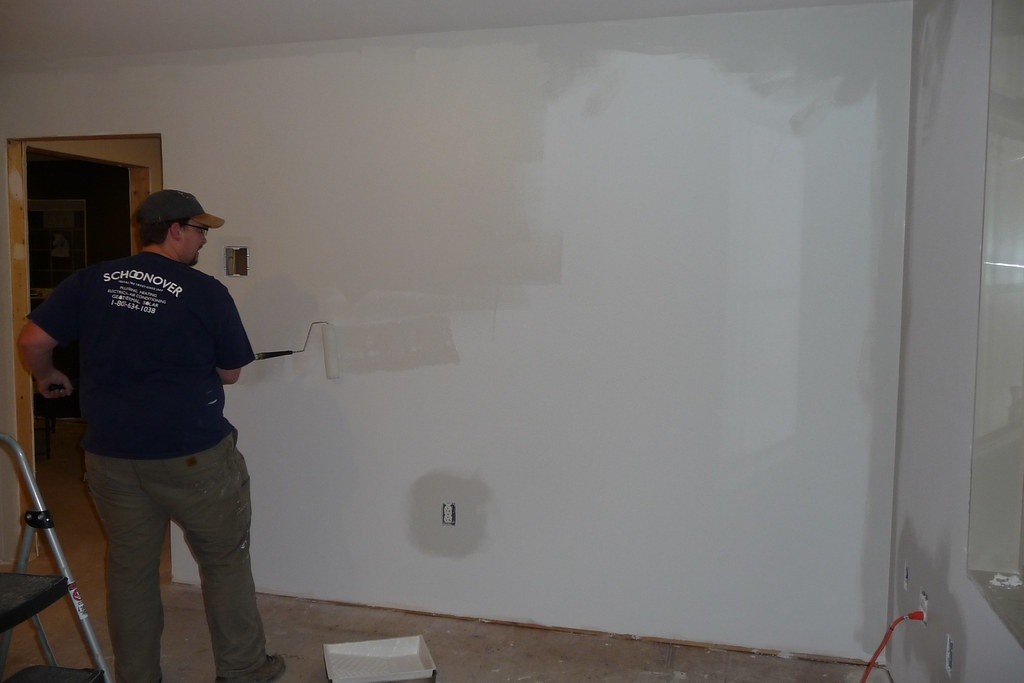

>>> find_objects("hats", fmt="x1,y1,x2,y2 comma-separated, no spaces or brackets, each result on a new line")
136,190,225,229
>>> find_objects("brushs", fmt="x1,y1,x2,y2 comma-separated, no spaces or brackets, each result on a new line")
50,321,339,393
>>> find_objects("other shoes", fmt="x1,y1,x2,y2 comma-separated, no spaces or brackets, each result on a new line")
215,655,285,683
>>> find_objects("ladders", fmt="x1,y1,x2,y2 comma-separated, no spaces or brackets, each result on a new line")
1,432,114,682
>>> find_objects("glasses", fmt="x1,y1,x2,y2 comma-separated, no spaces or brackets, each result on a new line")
169,221,208,236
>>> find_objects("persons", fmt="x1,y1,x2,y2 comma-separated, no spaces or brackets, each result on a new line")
17,189,287,683
